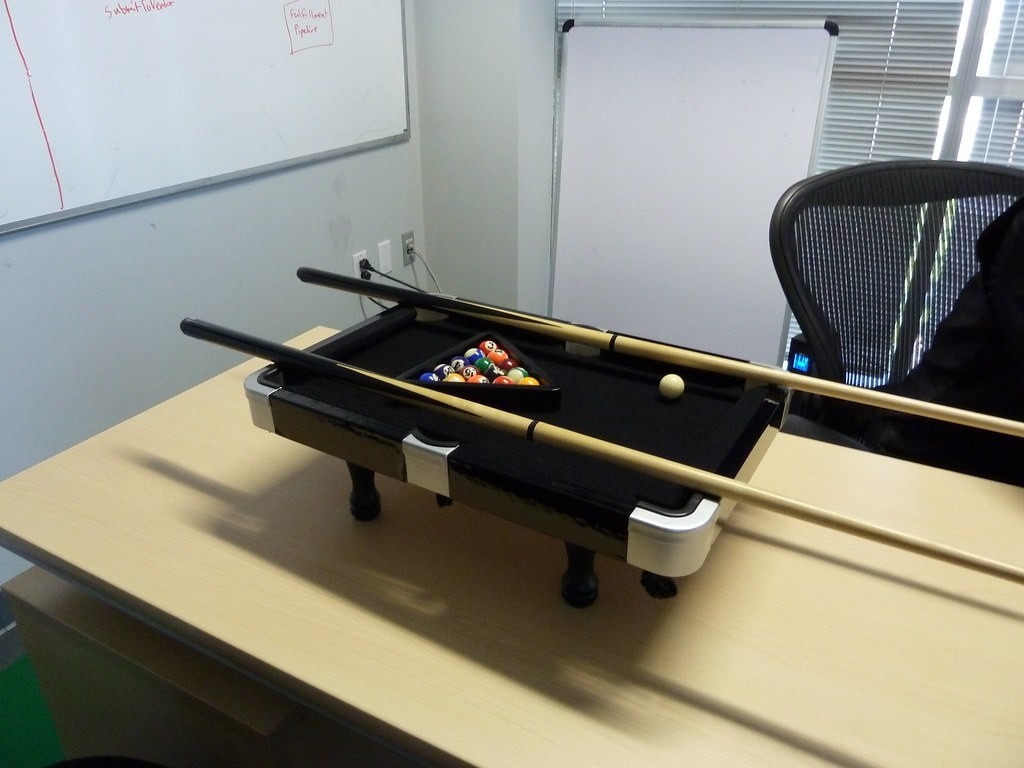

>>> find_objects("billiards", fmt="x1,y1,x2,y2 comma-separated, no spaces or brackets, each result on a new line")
421,338,539,388
659,372,685,399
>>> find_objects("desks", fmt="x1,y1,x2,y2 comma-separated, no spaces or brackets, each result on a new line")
0,325,1024,768
245,298,795,609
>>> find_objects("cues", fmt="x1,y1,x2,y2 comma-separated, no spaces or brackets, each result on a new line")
181,318,1024,585
296,268,1024,440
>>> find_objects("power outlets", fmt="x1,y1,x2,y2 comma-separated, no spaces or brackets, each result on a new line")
402,230,415,267
352,250,368,281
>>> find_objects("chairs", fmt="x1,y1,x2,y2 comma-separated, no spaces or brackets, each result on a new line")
769,158,1024,428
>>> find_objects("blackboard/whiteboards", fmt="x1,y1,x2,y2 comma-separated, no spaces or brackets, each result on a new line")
0,0,414,243
541,16,840,368
